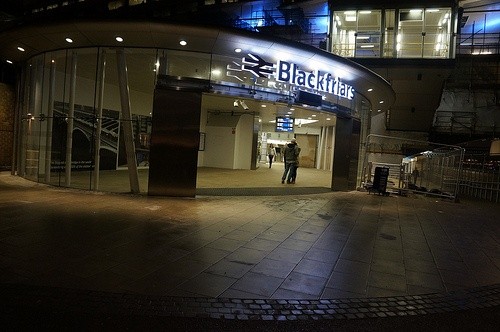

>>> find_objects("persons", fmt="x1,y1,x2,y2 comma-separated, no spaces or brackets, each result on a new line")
268,143,277,168
289,142,301,184
282,138,297,184
411,166,420,185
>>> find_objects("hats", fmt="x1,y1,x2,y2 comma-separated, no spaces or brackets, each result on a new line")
270,143,273,145
291,138,296,142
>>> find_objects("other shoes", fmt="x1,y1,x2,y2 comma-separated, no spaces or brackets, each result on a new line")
290,181,295,183
282,179,291,184
